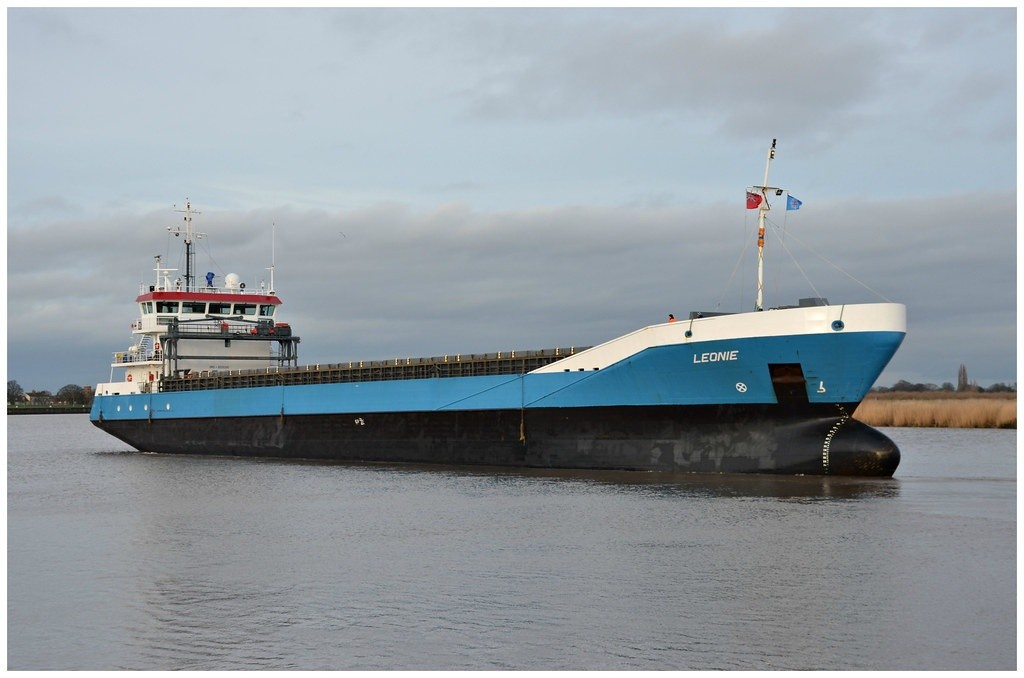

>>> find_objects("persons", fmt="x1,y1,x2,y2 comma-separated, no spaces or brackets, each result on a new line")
149,372,154,382
669,314,675,323
134,322,142,330
221,322,228,334
251,326,257,335
268,326,276,336
697,312,703,318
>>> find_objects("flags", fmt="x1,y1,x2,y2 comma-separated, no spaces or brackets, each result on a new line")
746,192,762,209
786,195,802,210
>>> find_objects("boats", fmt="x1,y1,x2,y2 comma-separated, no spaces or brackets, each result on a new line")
90,136,911,478
